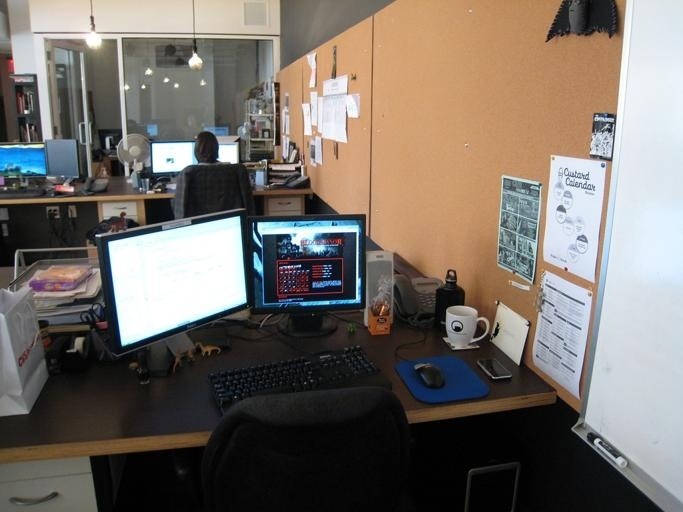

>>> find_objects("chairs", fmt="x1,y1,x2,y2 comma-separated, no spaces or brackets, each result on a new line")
183,163,255,217
198,388,416,510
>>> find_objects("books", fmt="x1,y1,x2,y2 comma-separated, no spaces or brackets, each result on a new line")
15,86,38,142
247,97,270,161
270,147,308,189
29,263,105,340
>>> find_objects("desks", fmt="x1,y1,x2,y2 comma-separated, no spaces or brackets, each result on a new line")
0,175,312,233
1,306,558,512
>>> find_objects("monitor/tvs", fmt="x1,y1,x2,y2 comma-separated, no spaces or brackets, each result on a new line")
96,206,255,377
216,143,240,164
44,139,82,190
150,140,199,182
202,125,230,135
247,214,367,337
98,129,122,156
0,142,48,186
146,124,160,136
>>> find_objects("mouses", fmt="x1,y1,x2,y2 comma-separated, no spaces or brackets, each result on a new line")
414,363,444,389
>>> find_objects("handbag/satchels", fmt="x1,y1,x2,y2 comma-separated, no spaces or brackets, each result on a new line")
0,287,50,417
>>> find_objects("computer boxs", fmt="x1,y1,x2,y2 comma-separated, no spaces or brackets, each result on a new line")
464,460,523,511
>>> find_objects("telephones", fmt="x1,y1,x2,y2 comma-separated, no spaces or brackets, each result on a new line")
83,177,109,193
393,273,445,326
284,171,310,190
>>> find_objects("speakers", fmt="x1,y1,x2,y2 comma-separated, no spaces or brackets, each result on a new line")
362,251,396,336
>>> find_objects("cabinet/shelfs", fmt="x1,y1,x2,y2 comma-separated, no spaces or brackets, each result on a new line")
243,98,277,162
8,72,42,142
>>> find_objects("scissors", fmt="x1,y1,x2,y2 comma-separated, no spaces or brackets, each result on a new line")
80,302,106,340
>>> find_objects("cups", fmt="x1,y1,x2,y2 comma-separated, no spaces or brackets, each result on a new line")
444,306,490,347
141,179,150,193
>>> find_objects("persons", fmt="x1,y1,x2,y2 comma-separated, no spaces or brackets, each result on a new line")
192,131,221,166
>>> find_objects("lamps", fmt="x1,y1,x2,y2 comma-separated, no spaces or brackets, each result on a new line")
84,0,101,50
186,1,203,73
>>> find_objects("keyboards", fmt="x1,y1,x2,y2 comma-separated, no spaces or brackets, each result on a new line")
206,343,393,419
0,188,45,198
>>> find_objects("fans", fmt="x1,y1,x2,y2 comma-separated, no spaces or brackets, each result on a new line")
115,134,151,184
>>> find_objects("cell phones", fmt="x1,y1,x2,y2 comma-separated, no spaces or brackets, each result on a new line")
477,357,513,381
